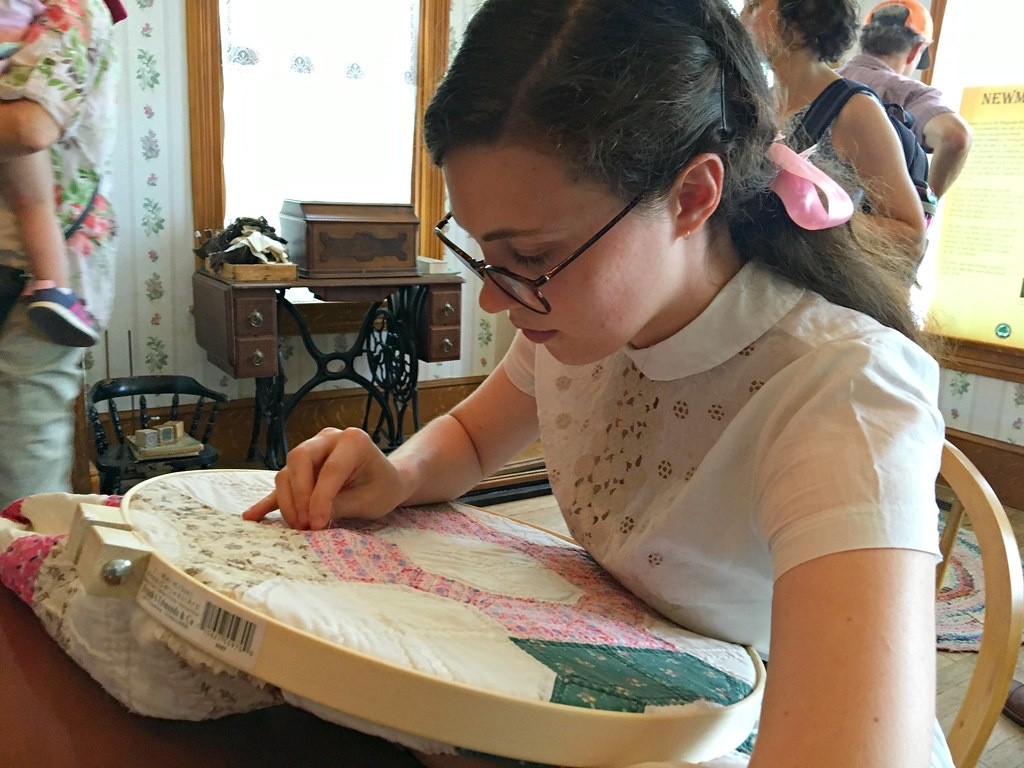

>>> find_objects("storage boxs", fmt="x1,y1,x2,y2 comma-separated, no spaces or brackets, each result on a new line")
277,196,420,280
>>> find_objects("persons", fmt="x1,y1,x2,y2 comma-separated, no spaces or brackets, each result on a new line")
733,0,929,298
1,0,120,507
822,0,972,215
241,0,976,768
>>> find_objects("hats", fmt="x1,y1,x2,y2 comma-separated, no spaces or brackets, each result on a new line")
865,0,933,70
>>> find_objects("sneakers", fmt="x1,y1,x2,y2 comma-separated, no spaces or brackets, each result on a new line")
22,279,101,348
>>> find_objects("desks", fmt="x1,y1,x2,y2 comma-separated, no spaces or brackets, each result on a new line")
0,561,549,766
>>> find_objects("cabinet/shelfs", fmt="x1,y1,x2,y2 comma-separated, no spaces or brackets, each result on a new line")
189,263,468,472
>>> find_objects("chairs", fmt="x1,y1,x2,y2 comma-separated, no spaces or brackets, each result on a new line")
930,436,1024,767
87,375,231,495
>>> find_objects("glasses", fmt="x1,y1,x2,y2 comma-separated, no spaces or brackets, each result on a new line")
435,178,659,315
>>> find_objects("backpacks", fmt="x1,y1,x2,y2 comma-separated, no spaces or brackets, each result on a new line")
788,75,938,234
